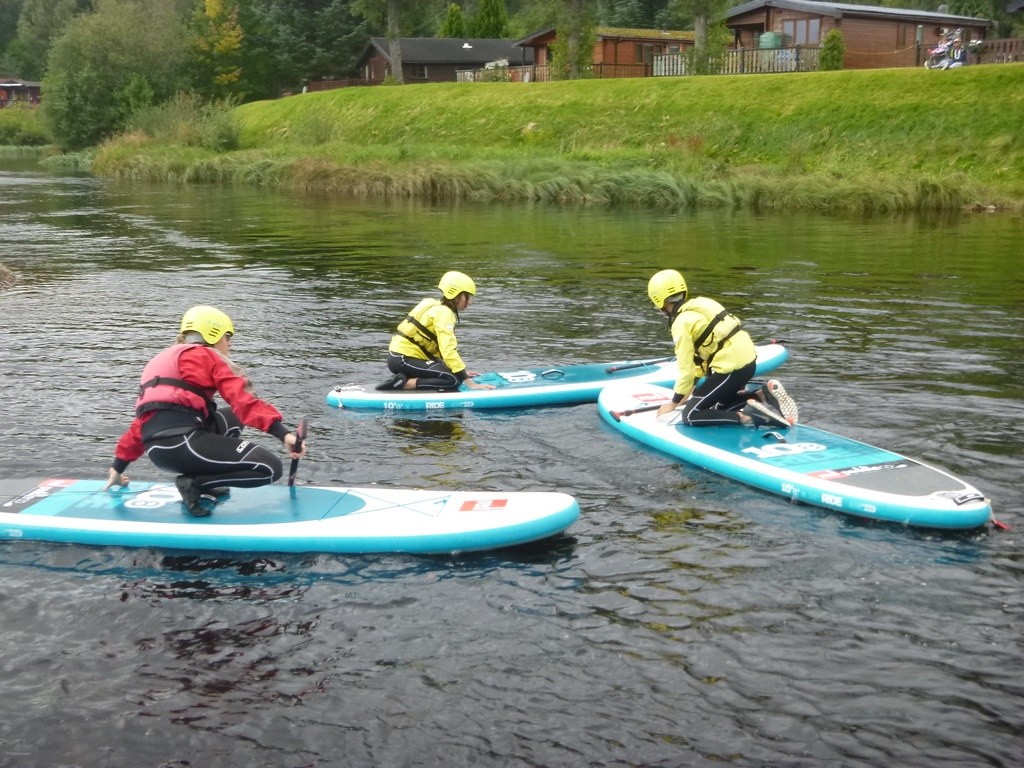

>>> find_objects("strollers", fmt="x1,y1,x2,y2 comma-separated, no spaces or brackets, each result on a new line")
924,27,964,70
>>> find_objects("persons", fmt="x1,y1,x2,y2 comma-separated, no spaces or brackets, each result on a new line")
103,305,307,517
648,269,798,428
924,36,966,70
374,271,497,391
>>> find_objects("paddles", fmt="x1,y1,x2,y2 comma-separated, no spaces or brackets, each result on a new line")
287,419,308,490
603,354,671,375
607,403,665,424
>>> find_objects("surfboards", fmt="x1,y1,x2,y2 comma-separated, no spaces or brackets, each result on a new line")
596,381,993,531
1,474,583,560
325,341,790,412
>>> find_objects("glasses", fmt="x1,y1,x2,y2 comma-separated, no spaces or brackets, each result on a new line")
226,333,232,342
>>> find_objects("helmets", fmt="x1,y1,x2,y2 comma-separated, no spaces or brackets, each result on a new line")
180,305,235,344
648,268,688,308
438,270,477,300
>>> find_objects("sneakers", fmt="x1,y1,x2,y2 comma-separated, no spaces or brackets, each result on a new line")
744,399,790,429
175,473,211,517
762,379,799,424
203,486,230,496
374,371,409,390
